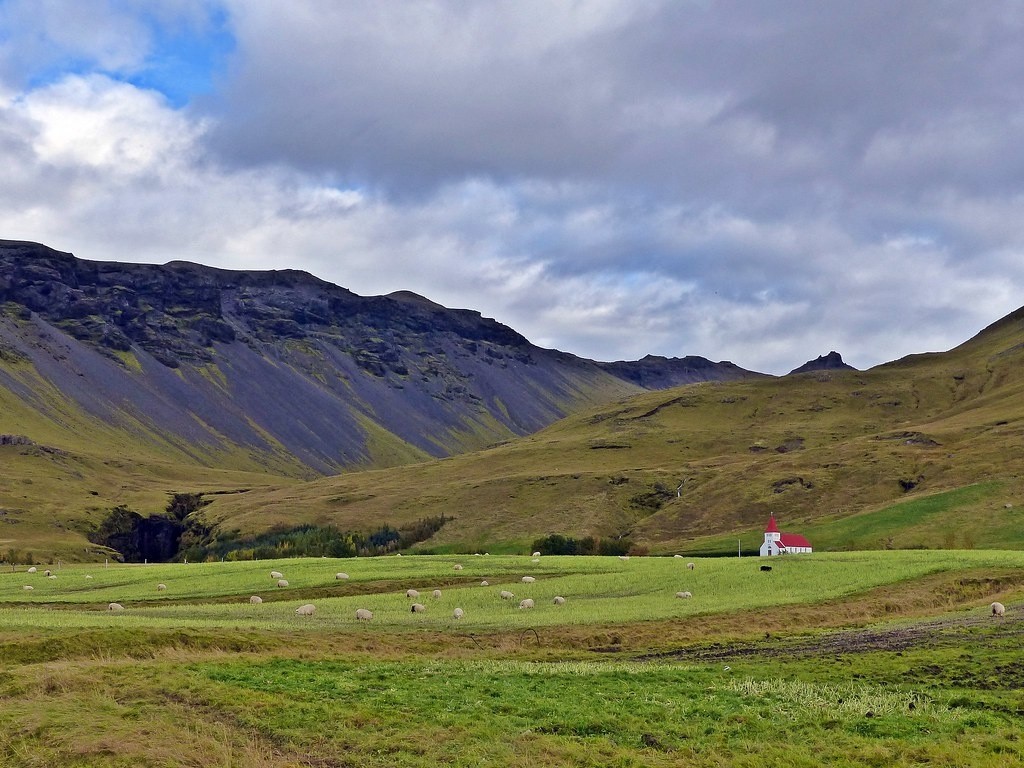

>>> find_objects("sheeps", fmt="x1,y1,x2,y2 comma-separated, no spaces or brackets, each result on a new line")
336,573,349,579
433,590,441,598
553,597,565,605
108,584,166,610
356,609,372,619
990,602,1005,617
22,567,57,590
251,571,315,615
532,552,540,556
501,591,514,600
519,599,534,609
676,592,692,599
687,563,695,570
454,565,462,570
522,576,535,584
619,556,629,561
674,554,683,558
407,590,426,613
453,608,463,620
481,581,489,586
